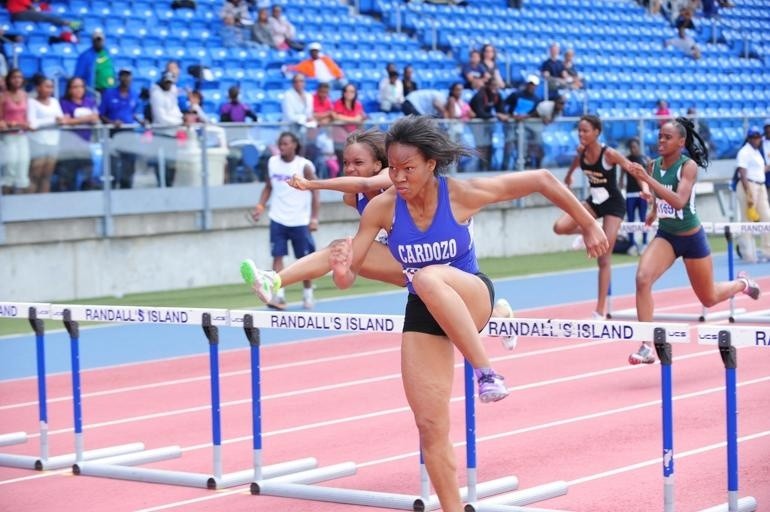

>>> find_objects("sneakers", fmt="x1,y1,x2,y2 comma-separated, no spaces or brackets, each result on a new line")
629,344,655,364
303,295,313,307
474,366,509,403
496,299,517,349
241,259,281,304
737,270,759,300
268,297,286,309
591,311,600,320
573,234,583,251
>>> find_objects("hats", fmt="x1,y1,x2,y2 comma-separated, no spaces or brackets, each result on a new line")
309,44,321,50
748,127,759,137
162,71,174,82
93,32,104,40
528,75,539,85
120,68,130,74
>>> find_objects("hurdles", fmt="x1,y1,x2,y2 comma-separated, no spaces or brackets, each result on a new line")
0,303,144,471
241,311,520,510
595,221,748,323
715,223,770,324
64,300,318,488
463,312,755,512
696,324,770,511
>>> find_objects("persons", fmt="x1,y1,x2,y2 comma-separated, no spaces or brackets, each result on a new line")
329,113,610,512
252,4,365,145
508,0,522,9
639,0,736,59
1,0,271,196
552,99,770,366
248,131,319,311
241,126,517,351
353,0,383,23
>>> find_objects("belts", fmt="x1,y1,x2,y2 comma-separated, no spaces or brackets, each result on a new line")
747,179,765,185
